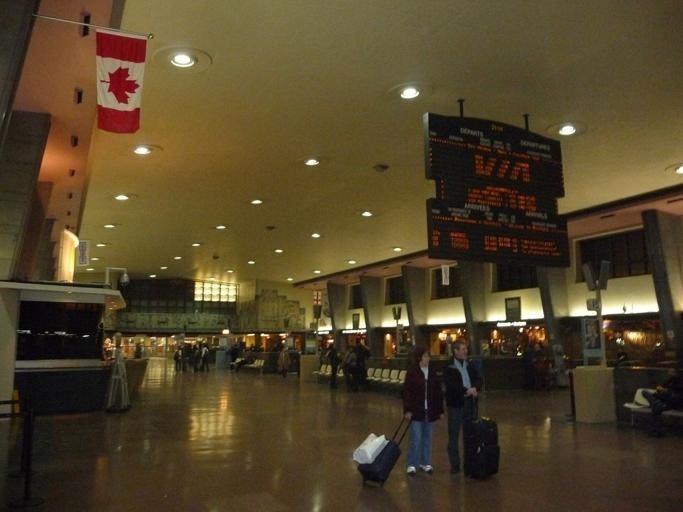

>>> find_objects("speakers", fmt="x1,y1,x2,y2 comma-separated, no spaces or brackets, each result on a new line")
581,261,597,291
598,260,610,290
392,307,397,319
397,307,401,319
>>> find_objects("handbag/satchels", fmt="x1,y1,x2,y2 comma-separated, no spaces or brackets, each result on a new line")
465,416,498,437
352,433,389,464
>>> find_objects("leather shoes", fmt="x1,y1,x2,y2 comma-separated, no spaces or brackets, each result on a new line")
451,467,461,473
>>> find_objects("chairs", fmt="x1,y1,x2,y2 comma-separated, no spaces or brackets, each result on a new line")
623,388,683,427
229,357,265,375
312,364,407,394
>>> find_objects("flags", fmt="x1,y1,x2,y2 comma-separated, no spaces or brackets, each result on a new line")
93,28,148,134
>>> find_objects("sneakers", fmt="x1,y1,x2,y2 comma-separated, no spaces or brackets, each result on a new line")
407,466,417,474
420,464,433,472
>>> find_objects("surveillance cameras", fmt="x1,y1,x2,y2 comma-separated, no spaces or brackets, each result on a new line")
120,273,130,286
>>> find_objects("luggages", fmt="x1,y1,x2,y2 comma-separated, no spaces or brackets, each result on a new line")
463,395,499,478
357,413,414,487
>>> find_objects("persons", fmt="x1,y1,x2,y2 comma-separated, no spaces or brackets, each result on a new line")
170,338,293,379
316,334,374,394
401,345,446,476
640,367,682,438
443,340,484,474
